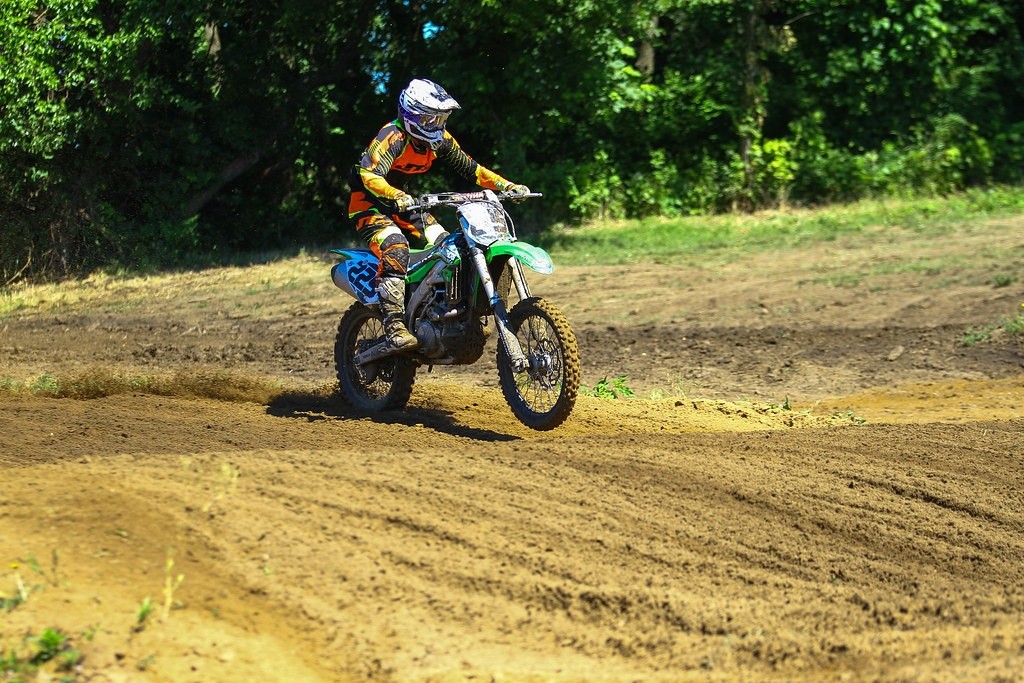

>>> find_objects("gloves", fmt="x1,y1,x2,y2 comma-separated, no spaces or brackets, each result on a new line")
394,194,416,215
506,184,530,205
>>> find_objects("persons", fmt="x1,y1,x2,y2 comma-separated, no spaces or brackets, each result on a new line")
349,79,529,350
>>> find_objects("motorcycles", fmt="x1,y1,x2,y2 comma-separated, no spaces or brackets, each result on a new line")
328,189,580,433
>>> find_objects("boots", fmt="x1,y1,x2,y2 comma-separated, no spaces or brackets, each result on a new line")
482,325,492,340
374,277,418,354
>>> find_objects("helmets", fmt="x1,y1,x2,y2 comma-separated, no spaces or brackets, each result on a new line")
398,78,462,151
